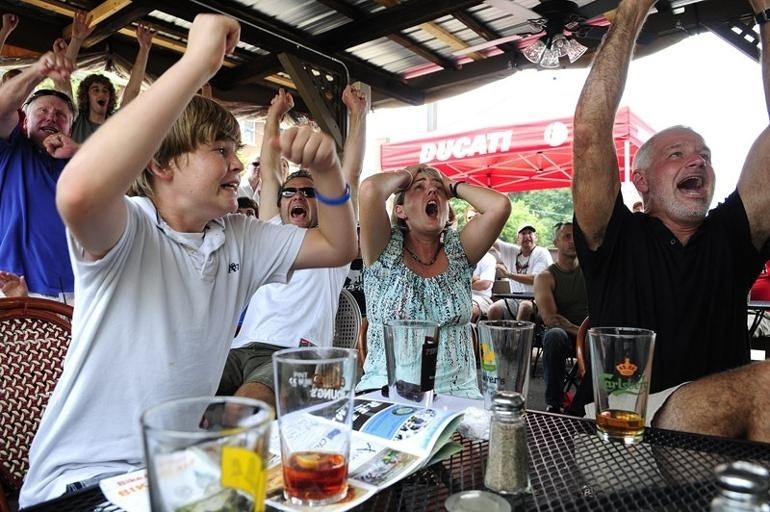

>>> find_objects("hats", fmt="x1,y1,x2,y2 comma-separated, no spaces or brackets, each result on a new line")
517,224,536,238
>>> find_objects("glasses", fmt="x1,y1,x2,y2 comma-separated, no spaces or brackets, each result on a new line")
252,162,260,168
277,186,317,208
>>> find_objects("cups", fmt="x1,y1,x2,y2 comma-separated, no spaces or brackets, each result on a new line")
271,348,357,506
475,319,536,418
382,319,443,411
588,326,656,445
141,395,271,512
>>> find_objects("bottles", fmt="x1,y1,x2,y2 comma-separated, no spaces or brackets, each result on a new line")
710,460,770,512
479,386,530,496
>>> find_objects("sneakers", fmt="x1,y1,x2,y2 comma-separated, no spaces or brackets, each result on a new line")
545,403,561,413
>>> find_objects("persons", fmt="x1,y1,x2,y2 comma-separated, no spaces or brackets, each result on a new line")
2,1,770,511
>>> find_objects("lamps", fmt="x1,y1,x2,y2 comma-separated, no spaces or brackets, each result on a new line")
523,1,588,67
